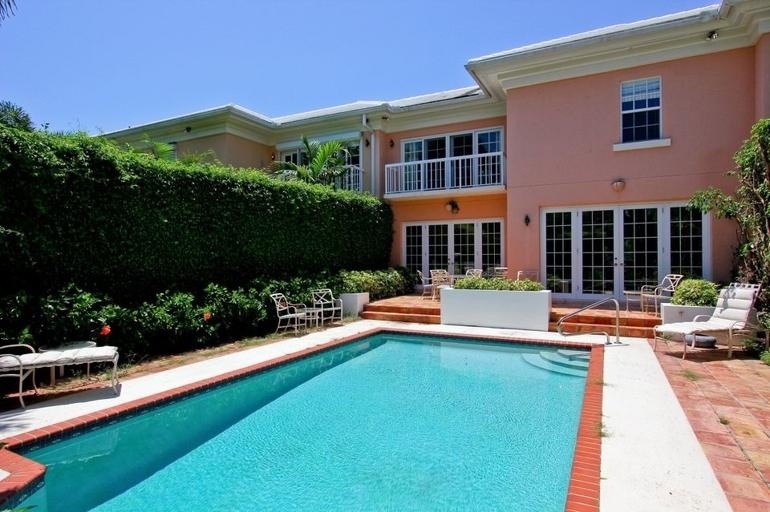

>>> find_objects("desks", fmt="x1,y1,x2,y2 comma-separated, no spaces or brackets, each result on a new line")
623,291,657,314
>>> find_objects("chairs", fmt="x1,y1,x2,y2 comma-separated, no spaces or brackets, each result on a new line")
416,266,509,303
653,281,769,360
269,288,343,336
641,274,684,317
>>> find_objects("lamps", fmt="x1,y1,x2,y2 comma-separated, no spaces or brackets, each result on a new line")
445,200,459,214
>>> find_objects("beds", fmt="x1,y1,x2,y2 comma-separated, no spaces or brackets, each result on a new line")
1,344,120,409
